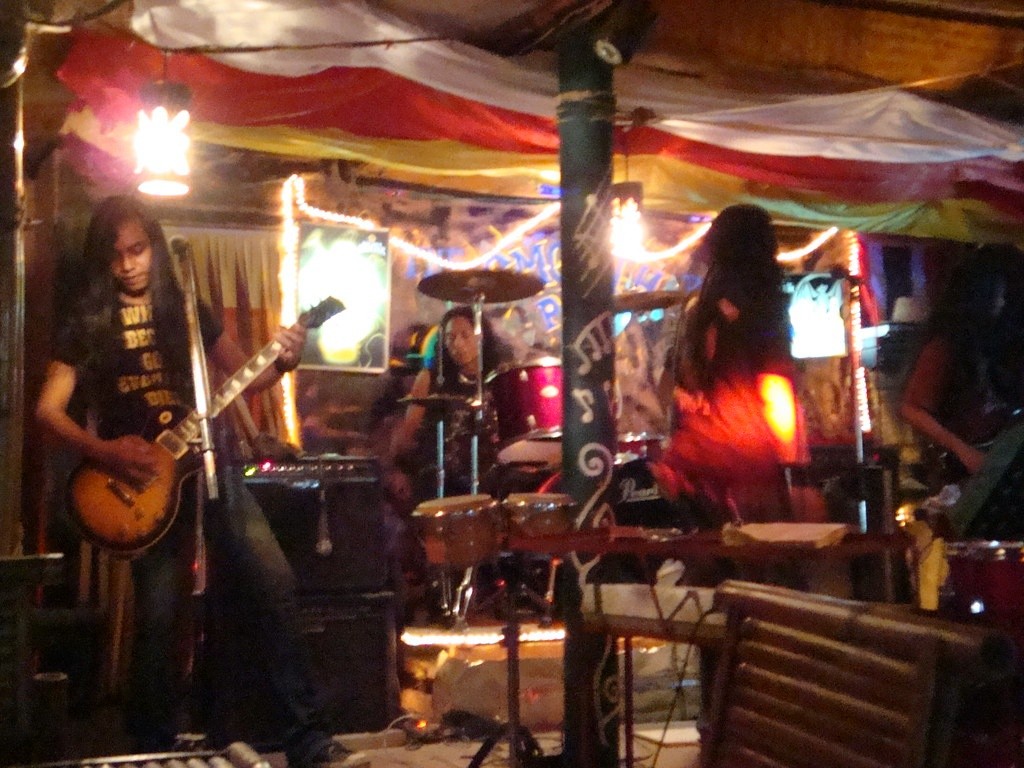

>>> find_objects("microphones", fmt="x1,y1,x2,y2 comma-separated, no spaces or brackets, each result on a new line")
171,237,190,255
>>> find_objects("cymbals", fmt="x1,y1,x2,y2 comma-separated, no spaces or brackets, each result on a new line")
395,394,470,409
417,269,547,304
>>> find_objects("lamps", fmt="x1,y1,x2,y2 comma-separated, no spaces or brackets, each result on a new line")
134,52,192,197
612,154,642,255
590,0,658,66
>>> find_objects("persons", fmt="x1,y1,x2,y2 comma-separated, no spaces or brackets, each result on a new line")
395,306,535,615
659,203,819,526
31,196,370,768
901,267,1024,543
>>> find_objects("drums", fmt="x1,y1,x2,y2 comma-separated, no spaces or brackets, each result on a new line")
507,491,573,557
415,493,499,565
902,538,1024,768
485,357,565,474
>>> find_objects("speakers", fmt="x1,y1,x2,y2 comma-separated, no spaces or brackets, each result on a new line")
203,455,388,597
201,596,402,750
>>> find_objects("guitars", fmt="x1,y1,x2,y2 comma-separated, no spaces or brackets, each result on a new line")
64,294,347,562
935,406,1024,487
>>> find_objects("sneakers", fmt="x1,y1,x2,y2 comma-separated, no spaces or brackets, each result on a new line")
290,740,371,768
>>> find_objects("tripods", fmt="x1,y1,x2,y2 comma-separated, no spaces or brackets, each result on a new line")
466,558,545,768
